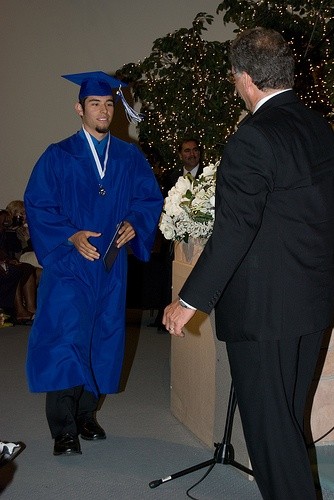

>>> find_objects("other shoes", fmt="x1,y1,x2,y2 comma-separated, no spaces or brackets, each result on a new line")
0,442,24,466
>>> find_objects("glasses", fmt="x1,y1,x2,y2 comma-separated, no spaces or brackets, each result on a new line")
226,68,252,84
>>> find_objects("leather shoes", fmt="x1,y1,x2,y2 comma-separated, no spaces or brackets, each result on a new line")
76,409,107,440
54,431,82,456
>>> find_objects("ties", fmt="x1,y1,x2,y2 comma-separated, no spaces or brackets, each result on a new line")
185,172,191,183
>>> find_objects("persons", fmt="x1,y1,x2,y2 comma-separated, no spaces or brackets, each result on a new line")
157,138,209,334
162,25,334,500
0,200,36,314
22,71,163,457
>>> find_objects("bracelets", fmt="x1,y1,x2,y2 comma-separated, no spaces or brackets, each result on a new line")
179,299,187,308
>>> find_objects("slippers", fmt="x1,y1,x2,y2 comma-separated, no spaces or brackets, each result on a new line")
15,314,35,325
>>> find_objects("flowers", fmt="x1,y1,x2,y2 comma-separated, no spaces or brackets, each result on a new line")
159,161,220,252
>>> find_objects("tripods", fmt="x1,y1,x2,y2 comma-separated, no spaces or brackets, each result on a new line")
148,386,255,489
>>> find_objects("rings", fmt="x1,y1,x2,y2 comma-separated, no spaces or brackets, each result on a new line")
170,328,174,330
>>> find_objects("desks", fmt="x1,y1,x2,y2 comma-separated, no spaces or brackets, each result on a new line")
171,234,334,448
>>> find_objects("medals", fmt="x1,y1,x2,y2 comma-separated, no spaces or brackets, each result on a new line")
99,189,106,197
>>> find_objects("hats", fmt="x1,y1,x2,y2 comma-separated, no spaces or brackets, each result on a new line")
61,71,147,125
